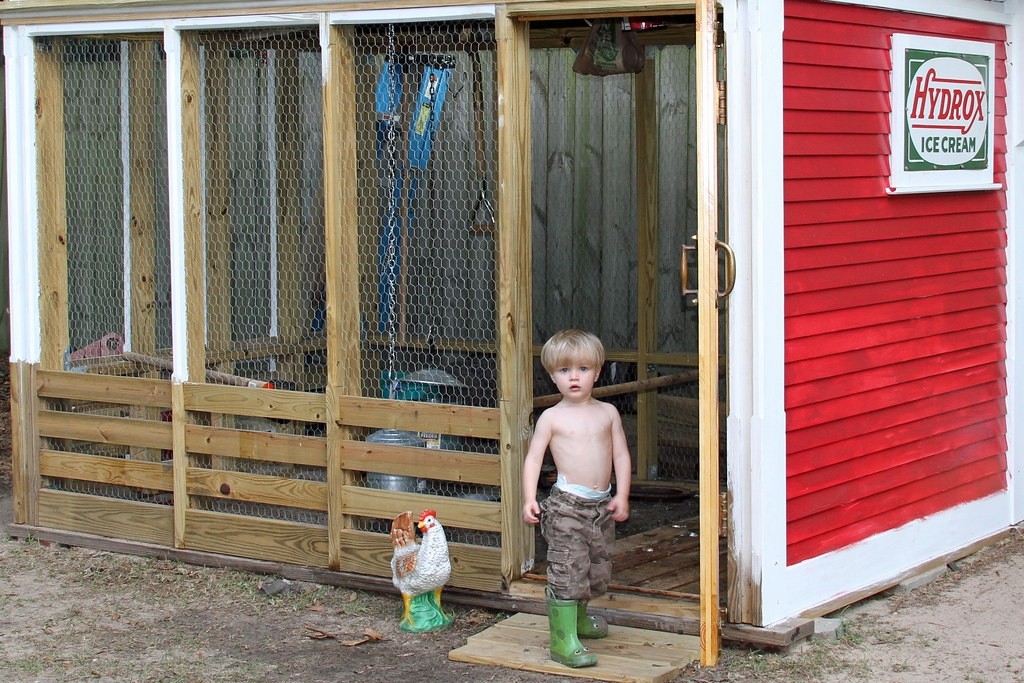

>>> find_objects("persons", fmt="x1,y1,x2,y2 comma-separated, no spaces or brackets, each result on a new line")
521,329,632,668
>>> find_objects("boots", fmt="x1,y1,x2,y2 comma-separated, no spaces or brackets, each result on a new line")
577,601,607,637
545,586,598,667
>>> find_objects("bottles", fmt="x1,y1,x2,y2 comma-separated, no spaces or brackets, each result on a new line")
364,426,424,500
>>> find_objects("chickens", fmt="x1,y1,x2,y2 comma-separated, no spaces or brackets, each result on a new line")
389,509,451,625
